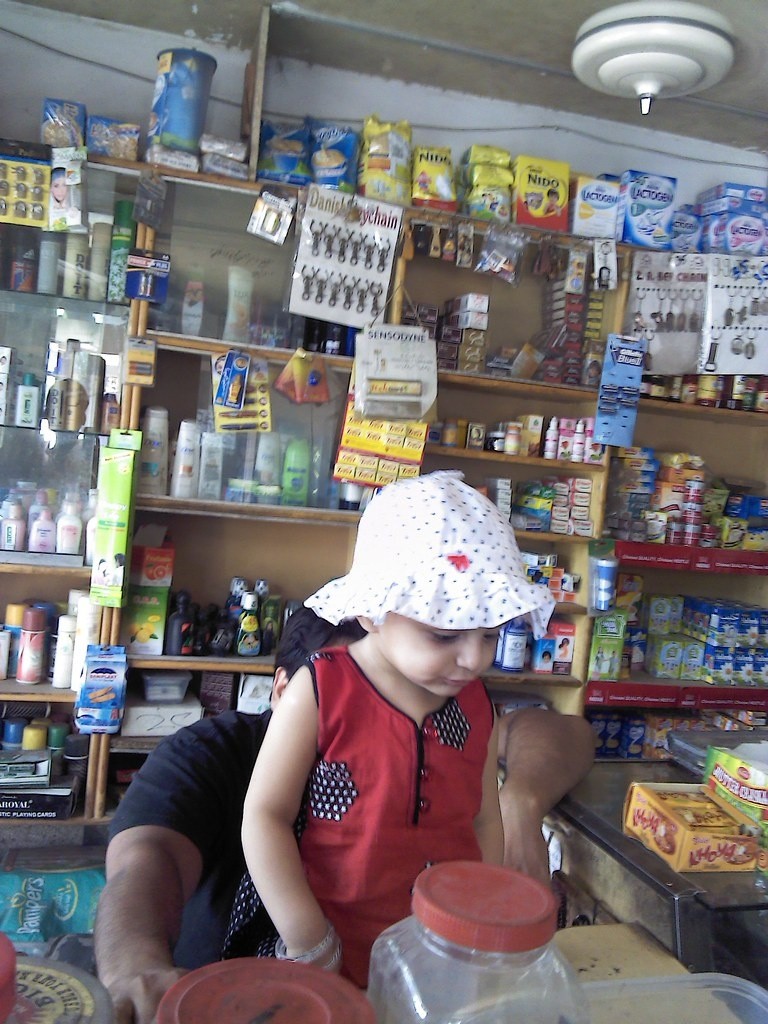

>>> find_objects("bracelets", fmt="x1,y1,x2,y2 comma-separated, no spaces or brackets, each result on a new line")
275,918,344,971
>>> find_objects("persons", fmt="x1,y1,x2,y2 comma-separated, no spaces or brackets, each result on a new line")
94,604,596,1023
49,167,71,210
240,471,557,992
536,651,553,671
544,188,563,218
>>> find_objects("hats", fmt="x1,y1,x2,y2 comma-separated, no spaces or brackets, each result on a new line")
301,470,555,641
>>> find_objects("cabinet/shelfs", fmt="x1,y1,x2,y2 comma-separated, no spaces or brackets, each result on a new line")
0,143,148,826
94,171,768,824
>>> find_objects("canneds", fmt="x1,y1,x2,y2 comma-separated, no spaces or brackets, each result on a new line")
666,478,717,549
641,372,768,413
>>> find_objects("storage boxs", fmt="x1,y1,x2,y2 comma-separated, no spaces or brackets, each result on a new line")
525,620,575,676
119,584,171,654
0,774,79,820
120,691,205,739
454,972,768,1024
623,781,759,873
404,262,608,386
236,673,276,714
484,475,592,537
142,667,192,703
200,671,236,720
131,539,175,589
586,410,768,762
91,427,142,608
704,740,768,877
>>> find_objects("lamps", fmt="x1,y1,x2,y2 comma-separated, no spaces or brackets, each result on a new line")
573,1,736,114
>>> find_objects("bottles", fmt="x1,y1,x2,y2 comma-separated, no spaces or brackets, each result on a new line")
543,416,560,461
638,375,768,415
367,860,586,1024
164,576,301,656
571,418,585,462
502,426,521,456
427,419,458,447
2,710,89,772
7,587,103,690
